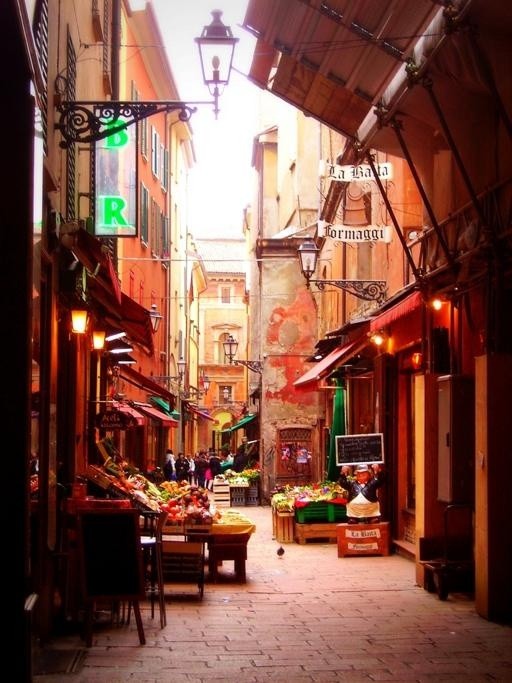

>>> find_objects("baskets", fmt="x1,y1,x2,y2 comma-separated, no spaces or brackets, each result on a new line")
89,457,113,489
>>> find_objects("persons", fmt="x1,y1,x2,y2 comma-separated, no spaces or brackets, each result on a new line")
162,434,251,492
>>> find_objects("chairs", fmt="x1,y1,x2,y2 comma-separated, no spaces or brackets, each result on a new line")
121,511,169,629
76,507,146,648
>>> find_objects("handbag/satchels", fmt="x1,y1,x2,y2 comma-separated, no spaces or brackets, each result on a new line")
163,461,173,480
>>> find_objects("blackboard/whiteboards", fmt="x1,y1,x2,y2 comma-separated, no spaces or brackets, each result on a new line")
335,433,385,466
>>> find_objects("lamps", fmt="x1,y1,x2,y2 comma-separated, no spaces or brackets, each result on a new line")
222,334,262,374
53,10,240,148
223,386,245,407
149,304,164,334
69,308,138,364
189,375,211,395
297,233,387,307
156,356,187,382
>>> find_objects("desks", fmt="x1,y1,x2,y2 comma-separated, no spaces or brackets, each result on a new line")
140,508,256,578
336,522,390,558
